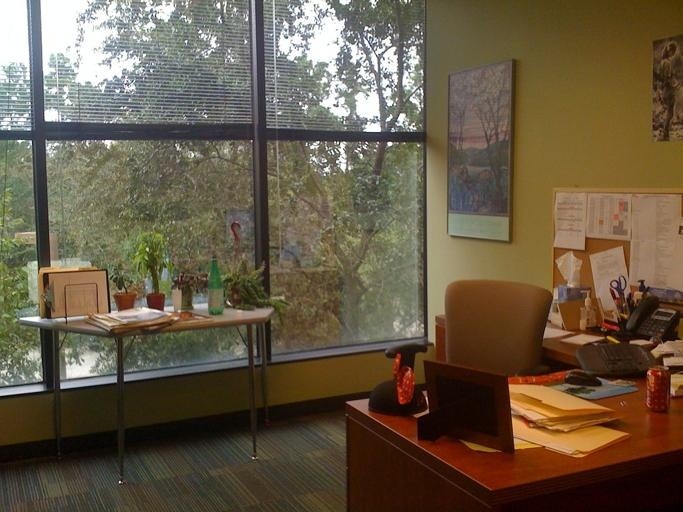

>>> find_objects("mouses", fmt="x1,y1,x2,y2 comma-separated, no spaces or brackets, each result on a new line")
564,371,602,387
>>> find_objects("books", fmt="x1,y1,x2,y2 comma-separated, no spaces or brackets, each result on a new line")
85,317,138,334
104,306,167,326
86,306,179,329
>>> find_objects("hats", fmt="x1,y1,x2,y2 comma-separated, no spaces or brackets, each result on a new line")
368,380,427,415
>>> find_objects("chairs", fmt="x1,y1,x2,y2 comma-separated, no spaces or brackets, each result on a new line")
445,278,552,375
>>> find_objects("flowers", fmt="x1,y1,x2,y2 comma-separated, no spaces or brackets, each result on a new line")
171,266,208,291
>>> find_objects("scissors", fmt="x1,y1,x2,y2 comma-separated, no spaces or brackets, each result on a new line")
610,275,627,302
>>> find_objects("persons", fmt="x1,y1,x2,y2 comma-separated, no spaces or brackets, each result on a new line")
655,41,681,142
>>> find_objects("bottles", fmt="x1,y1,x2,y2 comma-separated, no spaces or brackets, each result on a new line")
208,256,224,315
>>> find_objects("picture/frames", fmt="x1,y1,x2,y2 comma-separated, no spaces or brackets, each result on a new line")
445,58,517,242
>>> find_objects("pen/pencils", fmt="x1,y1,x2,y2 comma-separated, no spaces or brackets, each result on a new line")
609,288,633,314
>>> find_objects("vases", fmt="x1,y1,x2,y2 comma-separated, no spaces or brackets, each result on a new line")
171,287,194,312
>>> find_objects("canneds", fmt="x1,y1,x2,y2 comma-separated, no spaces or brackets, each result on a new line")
645,365,671,412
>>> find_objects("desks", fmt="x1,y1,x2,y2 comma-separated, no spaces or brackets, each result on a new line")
341,311,682,512
17,310,275,486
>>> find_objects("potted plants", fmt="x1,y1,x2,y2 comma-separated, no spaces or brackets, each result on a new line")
203,269,263,313
111,231,167,311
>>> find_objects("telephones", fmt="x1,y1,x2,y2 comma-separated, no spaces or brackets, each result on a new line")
626,296,680,340
